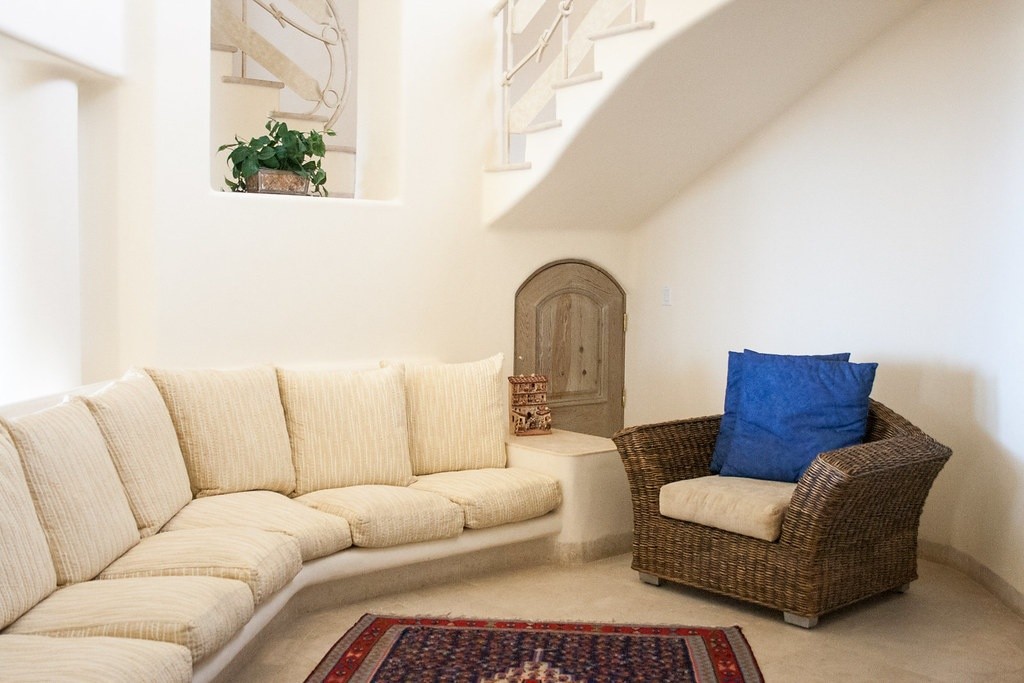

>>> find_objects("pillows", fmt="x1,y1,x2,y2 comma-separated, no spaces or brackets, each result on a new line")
2,395,142,586
708,351,851,473
378,351,507,476
143,362,297,498
719,348,879,483
77,366,194,539
0,422,58,631
273,361,418,497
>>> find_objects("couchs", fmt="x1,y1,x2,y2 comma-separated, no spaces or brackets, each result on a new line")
0,360,562,683
612,397,953,631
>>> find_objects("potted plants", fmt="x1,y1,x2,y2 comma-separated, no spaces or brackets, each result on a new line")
215,115,338,198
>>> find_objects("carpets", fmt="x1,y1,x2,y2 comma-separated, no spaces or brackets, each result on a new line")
303,613,766,683
